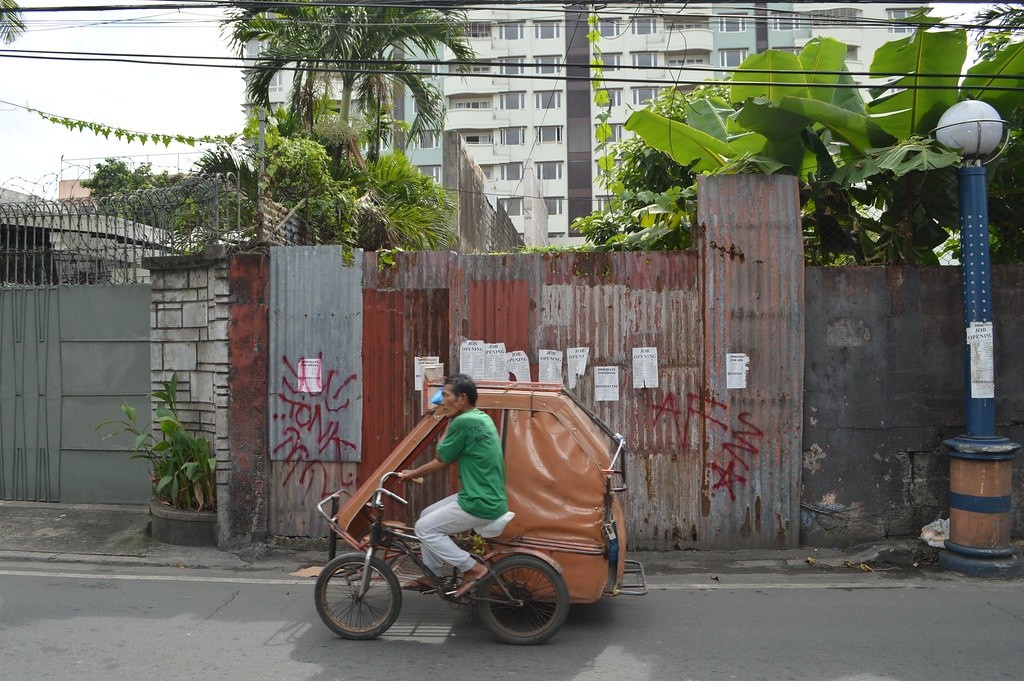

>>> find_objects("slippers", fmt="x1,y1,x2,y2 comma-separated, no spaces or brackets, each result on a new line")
452,569,494,600
401,580,436,591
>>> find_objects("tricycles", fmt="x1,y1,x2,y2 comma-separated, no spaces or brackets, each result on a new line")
314,381,647,643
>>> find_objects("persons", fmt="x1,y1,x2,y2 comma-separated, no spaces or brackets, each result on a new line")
399,373,507,600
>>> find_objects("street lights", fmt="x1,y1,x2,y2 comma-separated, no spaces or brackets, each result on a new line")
925,99,1022,578
